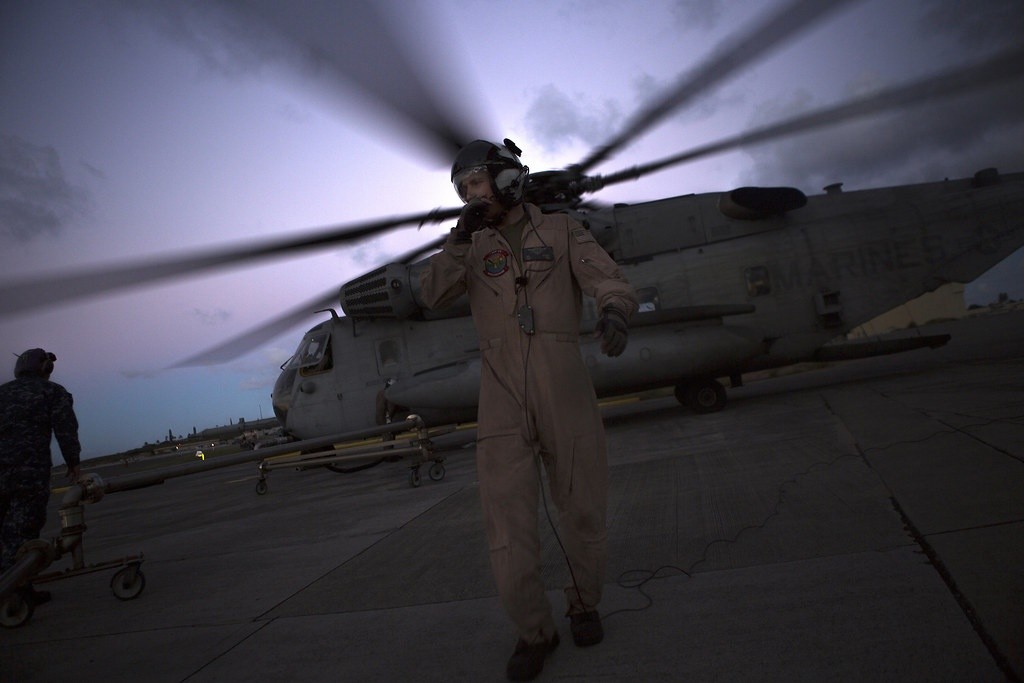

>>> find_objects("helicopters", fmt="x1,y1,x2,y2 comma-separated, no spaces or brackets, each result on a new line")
1,3,1024,468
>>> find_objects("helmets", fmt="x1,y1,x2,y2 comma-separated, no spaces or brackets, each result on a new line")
10,346,57,381
448,136,523,216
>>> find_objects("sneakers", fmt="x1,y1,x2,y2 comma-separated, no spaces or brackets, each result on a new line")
568,607,606,648
506,625,560,683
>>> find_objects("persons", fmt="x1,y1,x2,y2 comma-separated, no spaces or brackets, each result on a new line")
312,344,332,370
428,135,635,681
0,348,83,598
